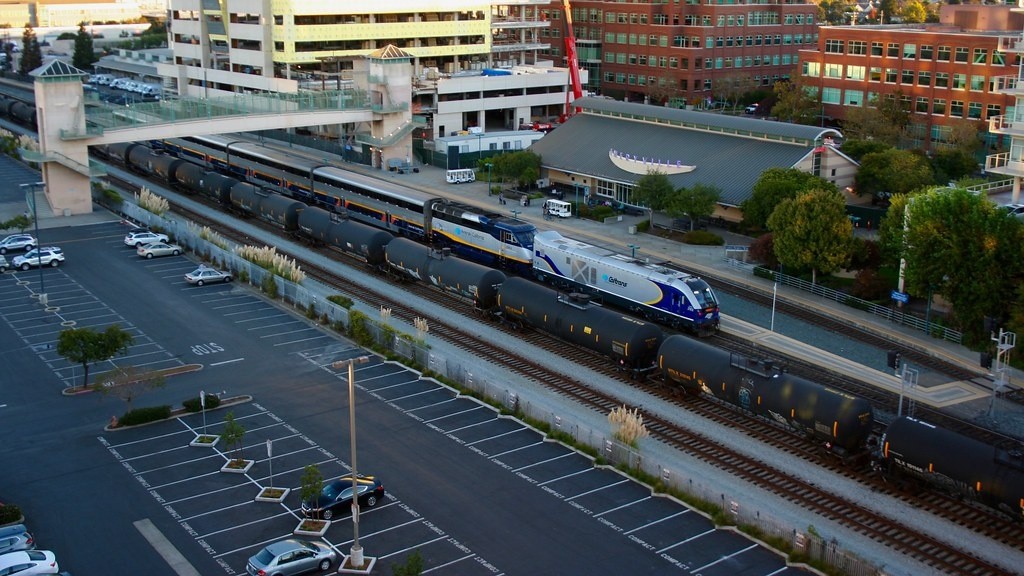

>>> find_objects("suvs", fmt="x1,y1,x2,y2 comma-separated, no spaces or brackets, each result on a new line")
124,229,170,249
11,246,65,271
0,234,38,255
745,104,759,114
1006,204,1024,224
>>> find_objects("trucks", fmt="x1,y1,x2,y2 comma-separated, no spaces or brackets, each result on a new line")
468,127,483,133
451,130,472,136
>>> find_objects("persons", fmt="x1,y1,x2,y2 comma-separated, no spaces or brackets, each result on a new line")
499,191,503,203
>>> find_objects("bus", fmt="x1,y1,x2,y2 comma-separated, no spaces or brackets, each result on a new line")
446,169,476,184
546,199,572,218
588,92,616,101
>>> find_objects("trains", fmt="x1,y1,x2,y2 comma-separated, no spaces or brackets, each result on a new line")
83,101,722,337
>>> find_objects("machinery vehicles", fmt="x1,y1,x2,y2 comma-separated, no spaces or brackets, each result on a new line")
534,0,584,136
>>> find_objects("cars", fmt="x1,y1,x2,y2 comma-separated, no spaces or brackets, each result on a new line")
301,476,385,519
0,255,9,273
88,74,160,95
0,523,65,576
245,539,337,576
136,242,182,259
184,264,235,286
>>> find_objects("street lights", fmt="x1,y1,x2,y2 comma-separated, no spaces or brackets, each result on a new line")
485,163,494,196
574,183,583,219
475,134,484,159
925,274,948,337
